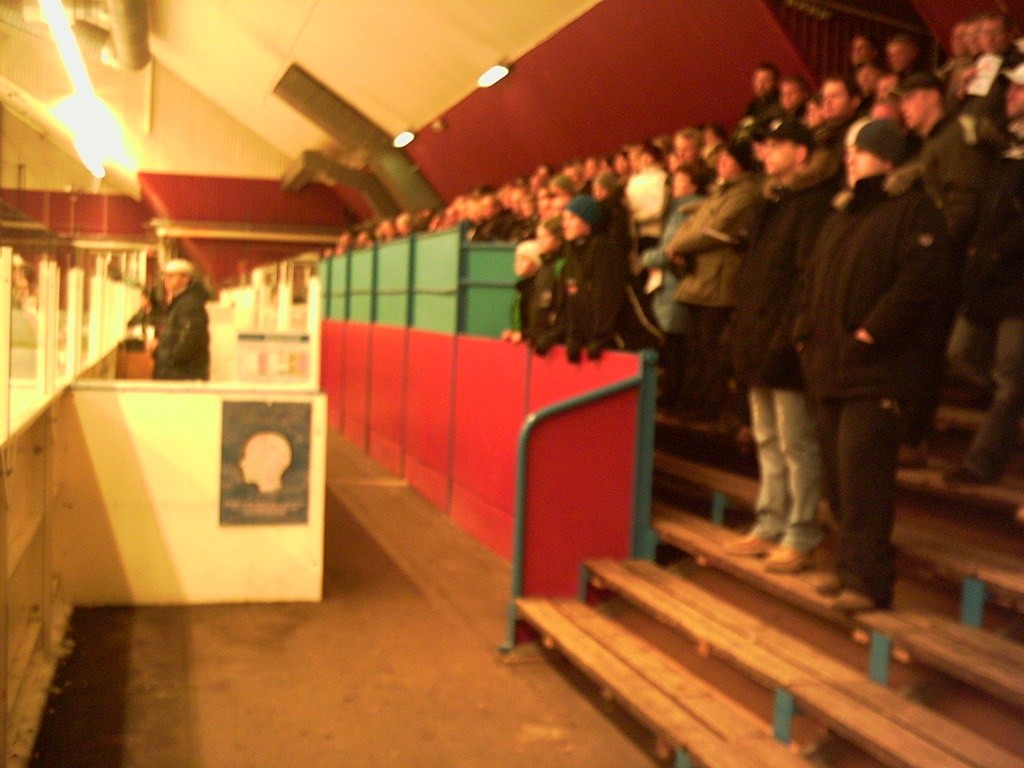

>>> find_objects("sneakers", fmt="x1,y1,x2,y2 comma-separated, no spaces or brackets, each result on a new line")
813,575,877,611
767,547,814,572
727,538,770,555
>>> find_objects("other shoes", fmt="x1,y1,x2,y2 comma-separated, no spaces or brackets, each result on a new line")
943,463,997,486
899,450,919,463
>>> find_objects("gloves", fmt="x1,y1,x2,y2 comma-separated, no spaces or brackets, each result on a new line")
588,342,601,358
567,346,580,362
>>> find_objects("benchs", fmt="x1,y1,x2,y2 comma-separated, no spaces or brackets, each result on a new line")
508,400,1024,768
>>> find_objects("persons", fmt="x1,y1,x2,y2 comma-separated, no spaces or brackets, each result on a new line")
324,15,1024,613
141,259,213,382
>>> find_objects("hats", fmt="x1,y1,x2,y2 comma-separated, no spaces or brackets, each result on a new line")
855,118,905,164
568,196,598,224
163,259,193,275
766,123,814,154
889,73,945,95
1004,63,1024,84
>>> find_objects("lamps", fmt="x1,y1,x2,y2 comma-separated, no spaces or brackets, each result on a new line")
477,65,510,89
392,131,415,149
